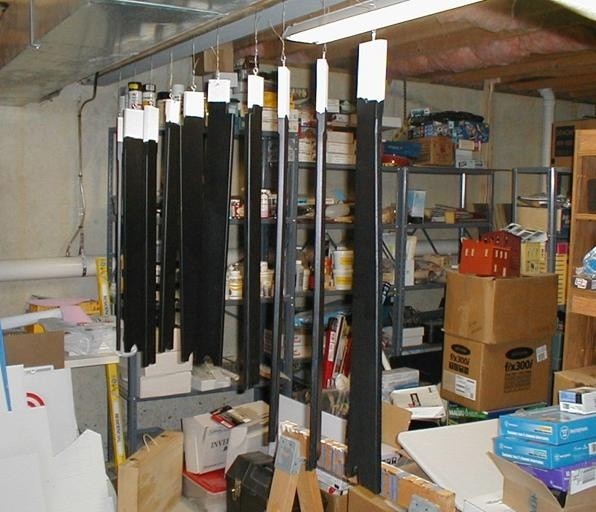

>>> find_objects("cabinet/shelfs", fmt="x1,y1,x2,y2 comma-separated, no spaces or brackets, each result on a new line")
554,128,596,405
155,135,572,418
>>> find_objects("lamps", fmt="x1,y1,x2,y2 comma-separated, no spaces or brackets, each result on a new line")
282,1,489,45
